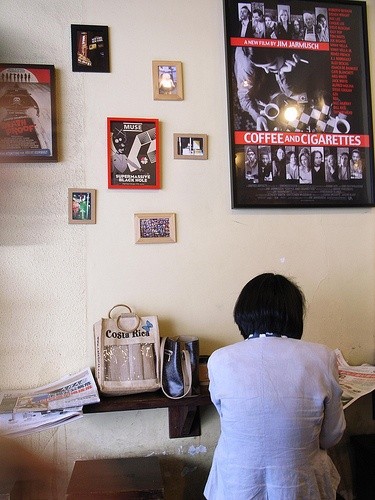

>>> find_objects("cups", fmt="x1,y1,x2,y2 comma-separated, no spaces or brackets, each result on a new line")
260,103,279,121
332,116,350,134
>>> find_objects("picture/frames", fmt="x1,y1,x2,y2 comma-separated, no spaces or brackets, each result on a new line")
0,62,59,164
221,1,374,210
151,60,185,102
67,187,97,225
173,133,208,160
134,212,177,244
71,25,111,73
105,117,163,190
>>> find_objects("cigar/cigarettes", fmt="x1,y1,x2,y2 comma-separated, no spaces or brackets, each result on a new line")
300,58,309,64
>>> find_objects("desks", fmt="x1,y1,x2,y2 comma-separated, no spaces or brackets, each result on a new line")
83,390,212,439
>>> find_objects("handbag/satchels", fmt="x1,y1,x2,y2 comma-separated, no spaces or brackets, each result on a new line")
160,334,199,400
93,304,161,395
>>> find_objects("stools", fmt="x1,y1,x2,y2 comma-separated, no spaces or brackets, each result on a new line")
65,455,167,500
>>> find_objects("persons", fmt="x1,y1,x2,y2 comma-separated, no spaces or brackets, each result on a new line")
203,273,346,500
246,147,362,183
159,73,175,95
234,46,308,131
239,6,328,42
140,218,170,238
